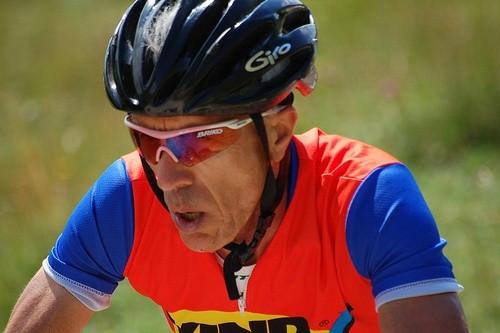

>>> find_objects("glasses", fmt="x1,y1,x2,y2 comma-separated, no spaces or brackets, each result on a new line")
122,104,284,168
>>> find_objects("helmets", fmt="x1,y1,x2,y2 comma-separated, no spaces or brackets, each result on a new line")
102,0,319,118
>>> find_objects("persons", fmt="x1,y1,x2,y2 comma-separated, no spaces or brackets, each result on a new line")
2,0,470,333
